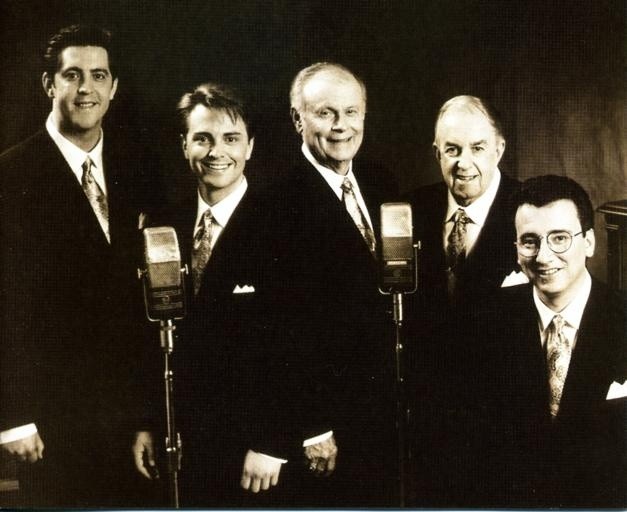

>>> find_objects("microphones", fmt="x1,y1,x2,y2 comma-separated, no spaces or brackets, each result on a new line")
379,202,416,294
143,226,186,320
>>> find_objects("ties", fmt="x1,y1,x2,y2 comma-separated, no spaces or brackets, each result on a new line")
448,209,473,272
340,177,377,259
82,158,111,244
192,210,216,298
545,315,572,420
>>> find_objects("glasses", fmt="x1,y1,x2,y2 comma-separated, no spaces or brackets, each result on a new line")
514,231,583,258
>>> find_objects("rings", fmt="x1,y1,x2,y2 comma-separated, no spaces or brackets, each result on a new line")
309,463,318,472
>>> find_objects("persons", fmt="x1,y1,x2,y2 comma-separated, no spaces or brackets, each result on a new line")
131,78,310,511
392,93,525,511
480,173,627,512
269,58,408,509
1,23,197,512
